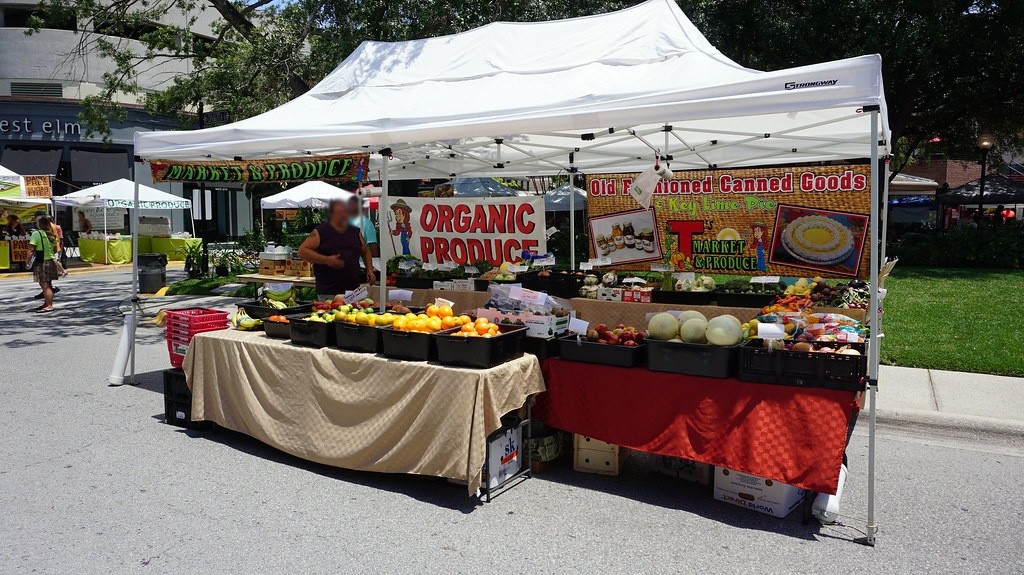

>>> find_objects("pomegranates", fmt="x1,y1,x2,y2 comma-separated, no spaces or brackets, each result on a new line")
586,324,646,346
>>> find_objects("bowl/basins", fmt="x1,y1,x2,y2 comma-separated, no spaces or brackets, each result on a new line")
261,317,291,339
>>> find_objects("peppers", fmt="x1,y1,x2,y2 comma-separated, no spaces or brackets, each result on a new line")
761,303,814,314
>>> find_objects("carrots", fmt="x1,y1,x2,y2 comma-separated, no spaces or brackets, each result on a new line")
775,292,815,308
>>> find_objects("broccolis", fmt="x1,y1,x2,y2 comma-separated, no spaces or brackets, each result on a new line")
578,275,603,298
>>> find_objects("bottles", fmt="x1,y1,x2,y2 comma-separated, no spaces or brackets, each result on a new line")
611,223,626,249
596,234,610,256
606,235,616,251
642,227,654,253
634,230,644,250
623,222,635,248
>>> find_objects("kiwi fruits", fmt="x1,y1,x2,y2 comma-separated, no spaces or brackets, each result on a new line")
535,307,567,318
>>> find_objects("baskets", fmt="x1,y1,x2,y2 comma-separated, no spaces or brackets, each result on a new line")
162,306,230,368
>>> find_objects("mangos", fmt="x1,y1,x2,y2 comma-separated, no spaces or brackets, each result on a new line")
326,294,435,314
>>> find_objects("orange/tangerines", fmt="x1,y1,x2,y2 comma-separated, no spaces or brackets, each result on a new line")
268,315,287,322
390,305,500,337
303,303,394,326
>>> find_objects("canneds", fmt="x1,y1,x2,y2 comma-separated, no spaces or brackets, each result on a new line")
595,227,655,256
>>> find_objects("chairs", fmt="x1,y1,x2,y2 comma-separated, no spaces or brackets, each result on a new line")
65,233,79,258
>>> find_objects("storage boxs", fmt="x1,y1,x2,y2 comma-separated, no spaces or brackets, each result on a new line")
163,253,871,518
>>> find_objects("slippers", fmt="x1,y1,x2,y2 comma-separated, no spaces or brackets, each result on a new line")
36,306,55,313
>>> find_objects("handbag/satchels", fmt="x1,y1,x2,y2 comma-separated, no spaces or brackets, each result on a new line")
34,250,45,265
28,255,36,269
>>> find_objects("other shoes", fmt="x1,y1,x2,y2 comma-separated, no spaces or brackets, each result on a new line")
62,271,67,278
53,287,61,294
34,291,45,298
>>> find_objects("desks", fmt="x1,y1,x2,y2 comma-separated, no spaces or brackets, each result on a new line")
571,297,866,332
0,240,31,269
532,355,859,535
78,238,203,265
237,273,318,301
183,329,546,505
367,285,487,315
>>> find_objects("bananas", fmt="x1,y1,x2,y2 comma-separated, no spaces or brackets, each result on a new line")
260,286,299,309
231,307,263,330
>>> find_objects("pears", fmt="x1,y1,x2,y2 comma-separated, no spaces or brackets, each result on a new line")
785,276,822,294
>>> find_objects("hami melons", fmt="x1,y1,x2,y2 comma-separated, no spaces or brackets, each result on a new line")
648,310,743,346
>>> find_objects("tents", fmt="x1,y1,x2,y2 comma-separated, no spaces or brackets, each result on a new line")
0,164,354,267
130,0,1024,548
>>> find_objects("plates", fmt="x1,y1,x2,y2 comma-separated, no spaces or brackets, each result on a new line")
782,228,855,265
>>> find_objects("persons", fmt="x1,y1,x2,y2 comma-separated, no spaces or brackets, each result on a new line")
972,209,980,225
1001,206,1015,222
347,195,378,277
3,212,93,313
298,197,375,306
990,205,1004,226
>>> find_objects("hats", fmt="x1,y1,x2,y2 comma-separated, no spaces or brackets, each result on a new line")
33,211,46,216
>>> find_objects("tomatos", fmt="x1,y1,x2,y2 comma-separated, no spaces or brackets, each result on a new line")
386,273,397,285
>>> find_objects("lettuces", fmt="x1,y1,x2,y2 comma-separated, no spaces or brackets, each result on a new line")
660,276,716,292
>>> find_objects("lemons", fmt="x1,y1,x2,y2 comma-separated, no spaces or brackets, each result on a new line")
740,320,796,340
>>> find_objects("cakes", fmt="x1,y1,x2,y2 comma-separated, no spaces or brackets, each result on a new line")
783,215,854,262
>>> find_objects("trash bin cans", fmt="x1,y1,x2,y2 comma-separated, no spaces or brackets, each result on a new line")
137,253,167,294
